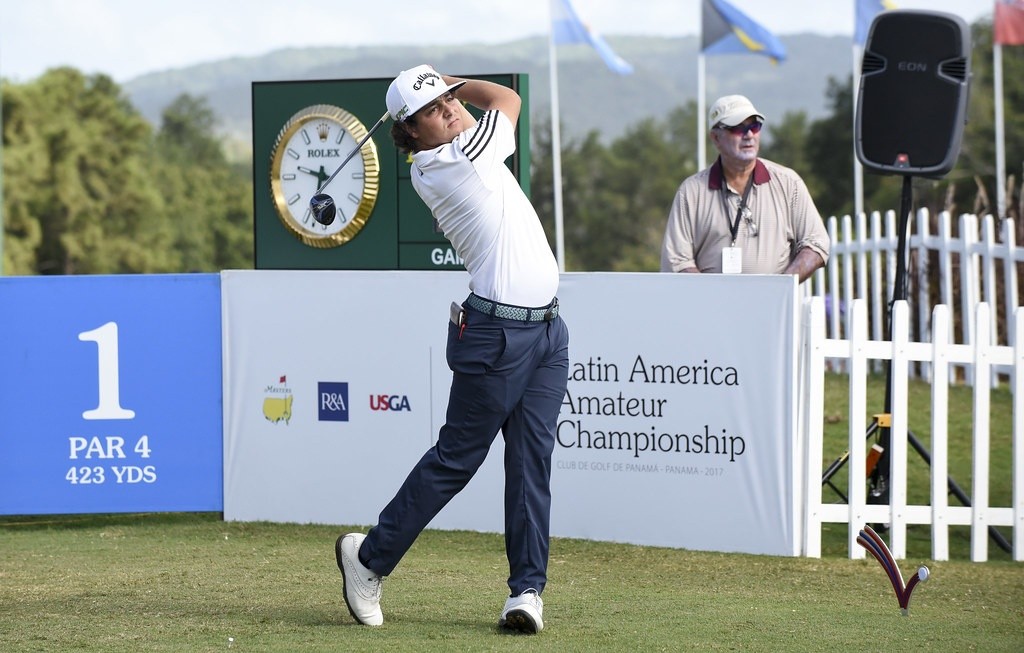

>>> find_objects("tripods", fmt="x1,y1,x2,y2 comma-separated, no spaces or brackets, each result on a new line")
822,178,1013,558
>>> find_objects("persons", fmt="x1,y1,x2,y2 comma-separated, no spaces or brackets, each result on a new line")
659,93,829,285
335,64,572,634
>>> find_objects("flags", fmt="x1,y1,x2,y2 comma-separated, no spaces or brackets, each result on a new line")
855,0,895,47
993,1,1024,47
701,1,785,64
551,0,637,74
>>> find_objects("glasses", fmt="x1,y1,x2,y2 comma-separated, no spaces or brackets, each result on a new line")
713,121,764,133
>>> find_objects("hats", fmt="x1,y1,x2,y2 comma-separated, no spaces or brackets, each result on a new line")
708,95,764,130
385,63,467,122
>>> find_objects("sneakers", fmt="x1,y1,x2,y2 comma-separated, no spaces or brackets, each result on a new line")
498,593,544,634
335,533,383,626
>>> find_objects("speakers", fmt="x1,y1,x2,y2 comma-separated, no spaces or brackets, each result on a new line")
851,8,971,175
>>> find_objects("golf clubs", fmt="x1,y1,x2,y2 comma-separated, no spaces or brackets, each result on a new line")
310,110,392,225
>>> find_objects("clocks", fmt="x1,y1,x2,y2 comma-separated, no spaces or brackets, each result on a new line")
267,102,381,249
